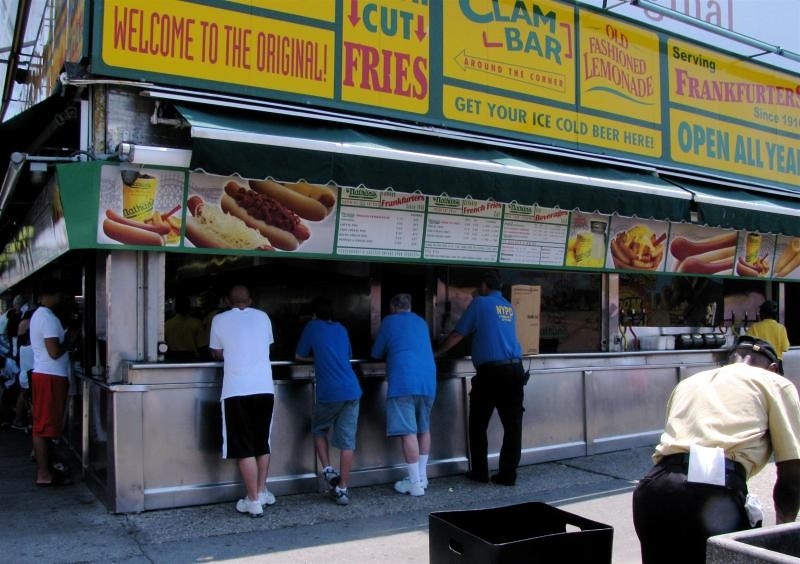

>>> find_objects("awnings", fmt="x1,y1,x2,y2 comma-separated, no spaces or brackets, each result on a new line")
171,103,800,237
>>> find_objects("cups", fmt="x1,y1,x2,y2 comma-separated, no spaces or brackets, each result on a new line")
122,176,156,223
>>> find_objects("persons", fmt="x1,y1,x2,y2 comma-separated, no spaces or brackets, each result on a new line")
371,293,436,497
434,271,524,486
0,266,82,487
633,335,800,564
209,285,274,515
744,300,790,376
165,290,280,360
723,280,765,329
295,299,363,505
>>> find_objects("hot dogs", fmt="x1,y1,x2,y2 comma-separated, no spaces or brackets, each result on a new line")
183,180,336,251
670,232,739,277
102,209,172,247
736,256,764,277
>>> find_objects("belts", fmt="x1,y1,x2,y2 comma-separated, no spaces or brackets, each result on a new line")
477,358,521,367
657,454,746,475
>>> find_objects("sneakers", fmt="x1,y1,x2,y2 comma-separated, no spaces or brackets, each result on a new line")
257,488,275,508
403,476,429,489
236,496,264,518
394,479,425,497
323,466,340,487
328,486,349,504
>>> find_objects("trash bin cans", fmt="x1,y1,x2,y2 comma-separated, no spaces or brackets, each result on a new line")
428,501,614,564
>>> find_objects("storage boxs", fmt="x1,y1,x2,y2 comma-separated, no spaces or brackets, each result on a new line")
428,502,615,564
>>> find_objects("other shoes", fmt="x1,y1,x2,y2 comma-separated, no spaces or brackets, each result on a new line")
12,422,31,430
491,472,515,486
466,470,489,483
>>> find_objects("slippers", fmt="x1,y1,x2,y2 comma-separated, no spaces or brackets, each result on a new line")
36,474,70,487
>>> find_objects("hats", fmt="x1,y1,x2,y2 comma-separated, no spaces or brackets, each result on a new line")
13,295,27,306
734,335,785,376
